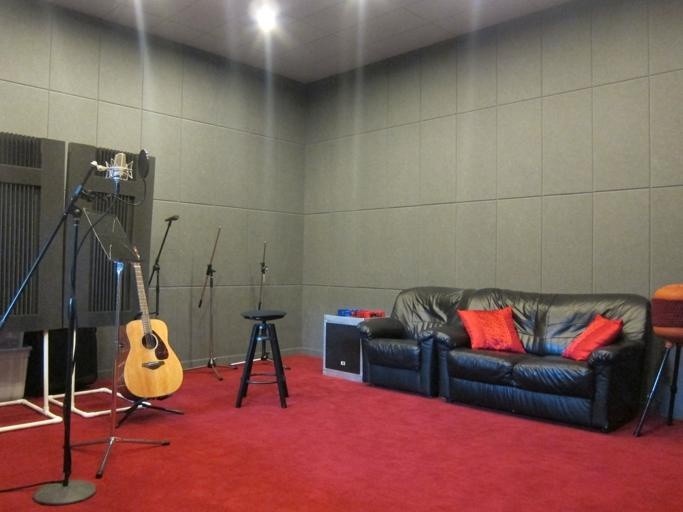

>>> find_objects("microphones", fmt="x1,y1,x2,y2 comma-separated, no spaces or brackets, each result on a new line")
112,153,127,195
164,215,179,221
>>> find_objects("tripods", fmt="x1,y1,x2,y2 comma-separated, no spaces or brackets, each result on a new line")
64,262,170,478
183,226,238,382
227,240,291,370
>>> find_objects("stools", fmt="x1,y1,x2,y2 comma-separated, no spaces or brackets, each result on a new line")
235,309,289,408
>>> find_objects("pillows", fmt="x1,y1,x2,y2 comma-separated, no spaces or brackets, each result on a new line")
456,306,528,355
651,298,683,327
562,314,624,361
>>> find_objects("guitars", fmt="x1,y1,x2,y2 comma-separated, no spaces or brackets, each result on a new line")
116,245,183,401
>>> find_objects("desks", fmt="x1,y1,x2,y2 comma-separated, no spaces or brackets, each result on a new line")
322,312,391,383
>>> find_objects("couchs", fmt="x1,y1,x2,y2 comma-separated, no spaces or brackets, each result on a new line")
435,287,651,434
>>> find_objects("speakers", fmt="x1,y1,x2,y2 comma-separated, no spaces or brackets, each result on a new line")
22,326,98,397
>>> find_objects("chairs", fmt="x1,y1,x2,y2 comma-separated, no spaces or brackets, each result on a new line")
634,284,683,437
356,285,465,397
